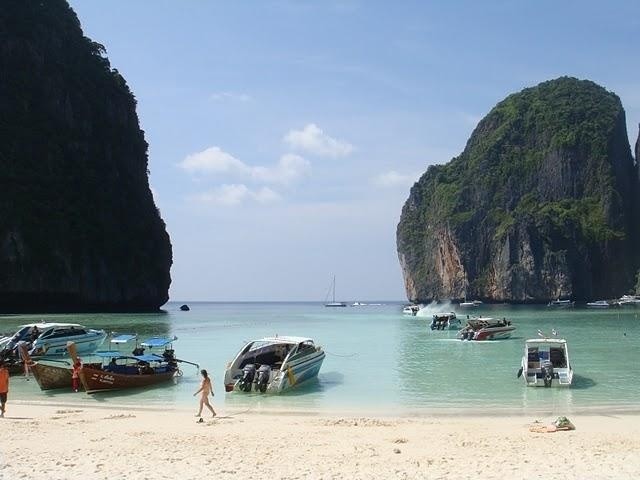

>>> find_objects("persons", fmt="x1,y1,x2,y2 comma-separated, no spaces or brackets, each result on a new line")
109,358,117,371
32,326,38,339
193,369,216,418
0,360,9,417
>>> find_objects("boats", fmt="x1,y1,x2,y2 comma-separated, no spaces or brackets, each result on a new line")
222,332,327,396
428,311,462,332
455,317,518,341
401,304,420,317
65,333,180,395
0,318,109,357
513,328,576,389
351,302,368,307
545,293,640,312
18,332,140,391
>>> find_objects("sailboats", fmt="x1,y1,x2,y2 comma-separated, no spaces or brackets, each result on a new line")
459,268,483,310
321,275,347,307
